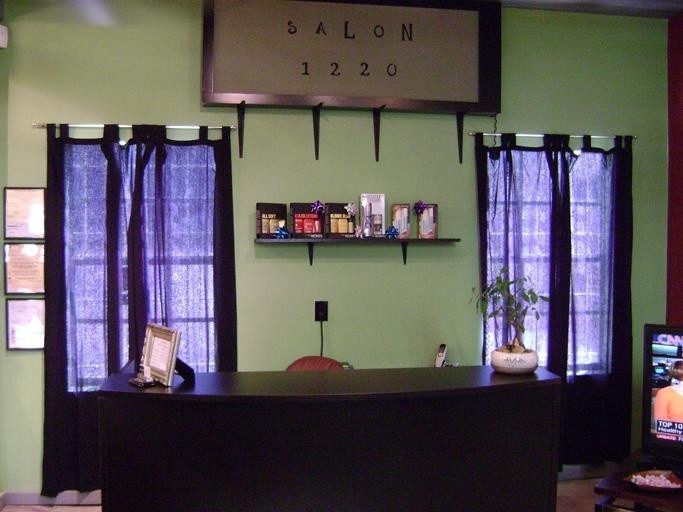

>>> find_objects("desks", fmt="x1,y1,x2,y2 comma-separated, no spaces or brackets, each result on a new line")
594,447,683,511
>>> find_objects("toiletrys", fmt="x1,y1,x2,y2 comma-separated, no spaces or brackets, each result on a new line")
257,202,434,240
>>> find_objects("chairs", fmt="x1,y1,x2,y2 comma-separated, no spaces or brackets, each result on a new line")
285,355,342,372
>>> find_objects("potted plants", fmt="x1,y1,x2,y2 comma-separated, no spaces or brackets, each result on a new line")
467,267,550,376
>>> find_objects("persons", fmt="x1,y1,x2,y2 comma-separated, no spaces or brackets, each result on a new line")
653,361,683,442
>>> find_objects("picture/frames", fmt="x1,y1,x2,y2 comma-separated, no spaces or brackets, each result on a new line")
137,321,194,387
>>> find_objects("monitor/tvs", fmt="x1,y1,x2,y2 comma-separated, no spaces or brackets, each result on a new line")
641,322,683,465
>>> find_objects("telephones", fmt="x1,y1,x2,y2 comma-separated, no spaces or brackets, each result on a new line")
433,344,447,369
